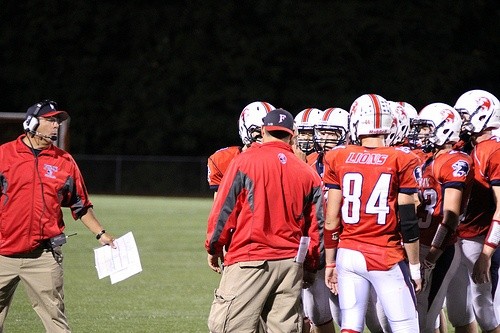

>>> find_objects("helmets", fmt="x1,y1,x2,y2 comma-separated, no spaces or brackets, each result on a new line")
293,109,324,152
454,90,500,135
349,94,392,141
387,101,418,145
315,108,350,141
414,102,461,146
238,101,277,144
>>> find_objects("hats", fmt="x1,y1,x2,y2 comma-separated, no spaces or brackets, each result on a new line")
264,109,294,135
26,104,68,121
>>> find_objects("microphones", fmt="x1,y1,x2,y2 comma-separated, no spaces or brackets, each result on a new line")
28,131,60,142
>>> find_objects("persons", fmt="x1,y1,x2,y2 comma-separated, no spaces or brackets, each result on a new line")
0,98,119,333
205,107,325,333
288,87,500,333
207,101,306,210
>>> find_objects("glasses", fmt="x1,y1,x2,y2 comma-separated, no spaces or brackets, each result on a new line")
38,117,63,126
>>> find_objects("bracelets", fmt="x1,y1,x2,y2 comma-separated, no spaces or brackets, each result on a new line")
95,229,106,240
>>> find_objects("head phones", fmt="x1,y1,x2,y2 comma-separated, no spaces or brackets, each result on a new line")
22,99,55,132
